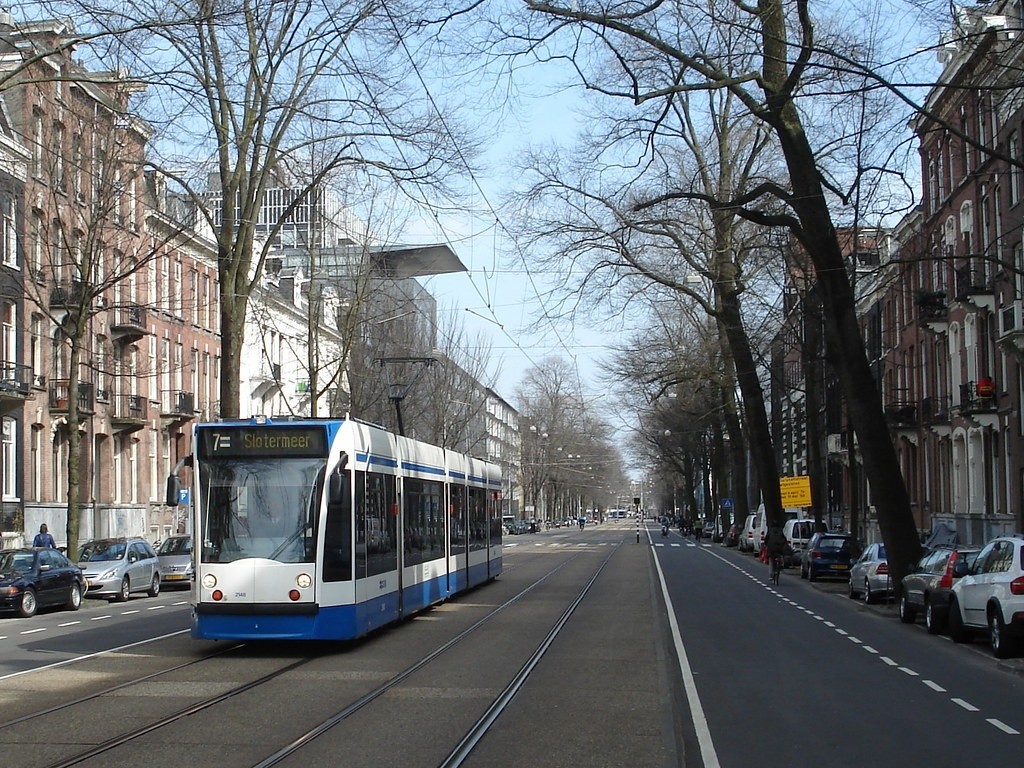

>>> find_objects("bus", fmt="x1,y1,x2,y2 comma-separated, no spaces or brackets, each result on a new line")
167,412,503,640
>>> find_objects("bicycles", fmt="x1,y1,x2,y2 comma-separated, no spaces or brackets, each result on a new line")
773,555,780,585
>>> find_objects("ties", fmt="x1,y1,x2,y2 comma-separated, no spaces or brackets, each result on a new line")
273,518,277,525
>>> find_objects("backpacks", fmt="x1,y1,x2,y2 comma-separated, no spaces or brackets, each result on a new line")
770,527,781,538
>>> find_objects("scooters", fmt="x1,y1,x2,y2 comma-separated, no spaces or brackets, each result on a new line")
580,521,585,532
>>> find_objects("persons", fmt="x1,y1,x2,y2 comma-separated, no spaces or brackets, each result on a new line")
579,515,585,531
255,501,290,538
677,515,703,540
530,514,536,534
33,524,55,549
653,515,675,531
764,520,790,580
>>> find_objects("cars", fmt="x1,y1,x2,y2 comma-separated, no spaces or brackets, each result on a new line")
0,534,191,617
503,517,593,535
692,503,1024,656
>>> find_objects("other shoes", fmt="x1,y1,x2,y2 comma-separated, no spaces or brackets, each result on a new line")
768,577,774,581
